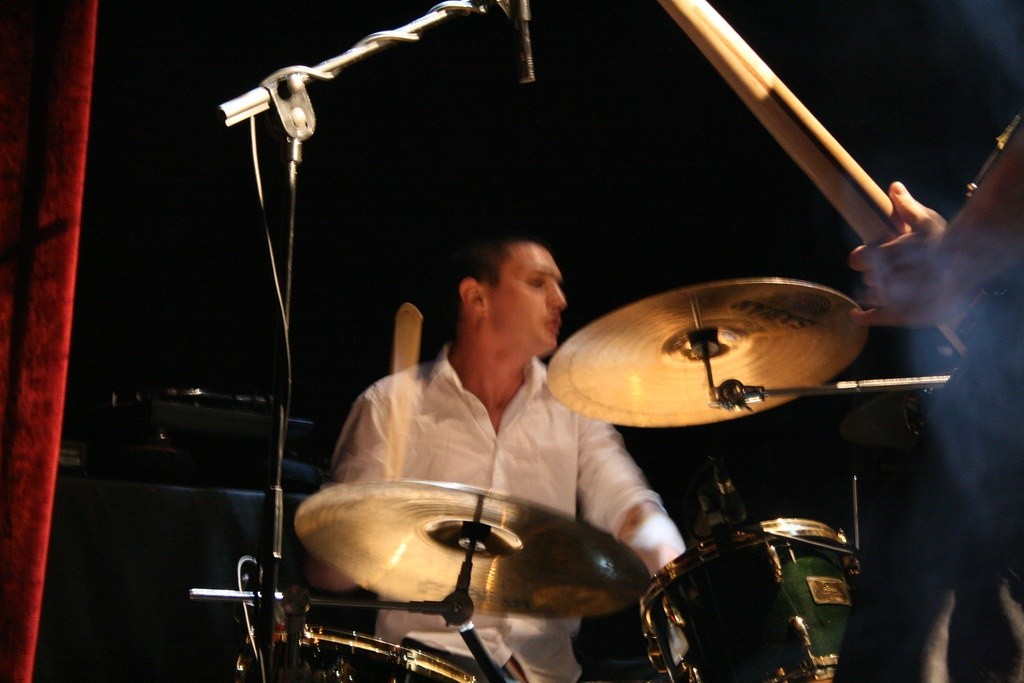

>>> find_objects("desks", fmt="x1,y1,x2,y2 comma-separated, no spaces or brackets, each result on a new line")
34,476,306,682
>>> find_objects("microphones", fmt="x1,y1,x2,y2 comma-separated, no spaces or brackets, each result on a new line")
511,0,535,83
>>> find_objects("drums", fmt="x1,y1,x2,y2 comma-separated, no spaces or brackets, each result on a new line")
237,625,480,683
638,513,858,683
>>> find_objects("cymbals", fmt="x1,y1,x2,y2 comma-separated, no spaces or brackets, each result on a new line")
292,477,653,614
546,277,875,429
839,388,922,450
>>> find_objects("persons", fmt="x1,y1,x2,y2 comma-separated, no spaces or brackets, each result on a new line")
849,118,1024,331
312,230,686,683
